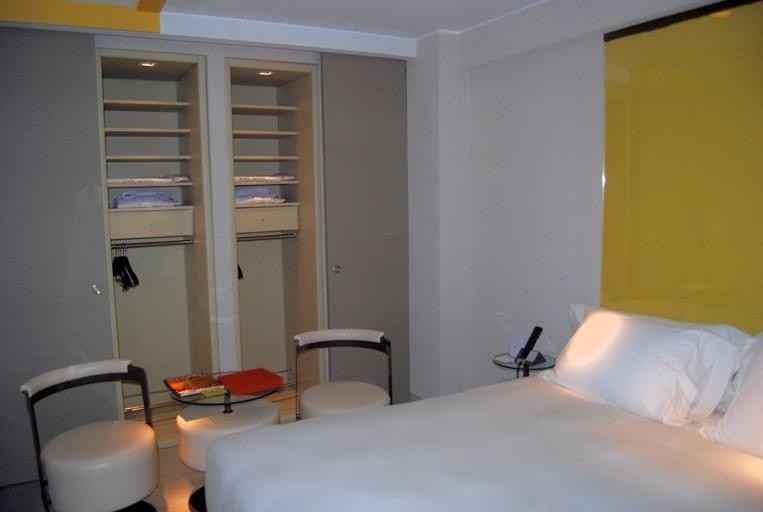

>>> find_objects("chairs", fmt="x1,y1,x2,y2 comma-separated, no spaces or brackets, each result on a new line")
19,358,162,512
293,327,392,419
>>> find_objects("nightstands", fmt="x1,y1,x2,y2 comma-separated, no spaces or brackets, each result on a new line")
492,351,556,376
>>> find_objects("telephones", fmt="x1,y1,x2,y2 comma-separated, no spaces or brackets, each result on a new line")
514,326,546,365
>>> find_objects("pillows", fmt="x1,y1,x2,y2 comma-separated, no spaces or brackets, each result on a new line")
538,301,763,459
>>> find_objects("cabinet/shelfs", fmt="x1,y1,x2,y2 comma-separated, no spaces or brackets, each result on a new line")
94,34,331,451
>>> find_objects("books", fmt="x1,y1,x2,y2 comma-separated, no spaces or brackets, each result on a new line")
163,372,224,397
198,372,229,397
215,368,285,396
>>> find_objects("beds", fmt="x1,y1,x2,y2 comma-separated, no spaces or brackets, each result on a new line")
205,377,763,512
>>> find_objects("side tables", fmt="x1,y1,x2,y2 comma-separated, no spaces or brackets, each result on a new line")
168,370,280,512
